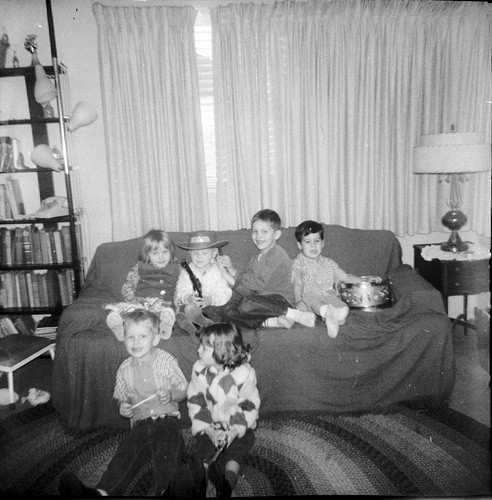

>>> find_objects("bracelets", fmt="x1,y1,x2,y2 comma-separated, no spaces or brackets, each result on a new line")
295,300,302,306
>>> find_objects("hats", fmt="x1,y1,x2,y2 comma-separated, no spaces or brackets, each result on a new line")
175,230,229,250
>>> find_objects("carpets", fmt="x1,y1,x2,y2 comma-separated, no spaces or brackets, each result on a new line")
0,395,492,500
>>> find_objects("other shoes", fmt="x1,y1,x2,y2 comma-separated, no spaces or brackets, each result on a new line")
184,302,215,327
159,307,176,340
105,312,125,341
176,312,198,337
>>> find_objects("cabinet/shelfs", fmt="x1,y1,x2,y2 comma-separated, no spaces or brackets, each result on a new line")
0,64,82,315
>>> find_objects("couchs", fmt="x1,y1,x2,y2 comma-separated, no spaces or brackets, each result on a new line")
52,225,453,434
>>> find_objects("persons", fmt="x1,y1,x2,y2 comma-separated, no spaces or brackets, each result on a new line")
217,210,294,328
186,322,262,498
288,221,382,338
57,309,189,500
105,228,180,340
172,229,232,332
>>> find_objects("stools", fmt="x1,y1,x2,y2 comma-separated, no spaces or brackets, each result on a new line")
0,332,57,410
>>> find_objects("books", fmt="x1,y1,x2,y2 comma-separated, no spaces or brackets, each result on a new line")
0,226,78,264
0,270,75,310
0,314,59,340
0,178,27,221
0,136,22,170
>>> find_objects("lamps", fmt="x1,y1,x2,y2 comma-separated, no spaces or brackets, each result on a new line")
414,129,491,254
31,0,98,299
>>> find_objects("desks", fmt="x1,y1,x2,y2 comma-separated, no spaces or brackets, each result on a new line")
412,242,492,335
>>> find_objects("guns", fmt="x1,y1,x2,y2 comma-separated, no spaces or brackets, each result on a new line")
181,256,202,299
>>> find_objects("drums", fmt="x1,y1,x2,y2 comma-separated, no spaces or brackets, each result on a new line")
338,276,395,310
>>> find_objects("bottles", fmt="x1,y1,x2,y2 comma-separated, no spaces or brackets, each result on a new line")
13,51,21,68
